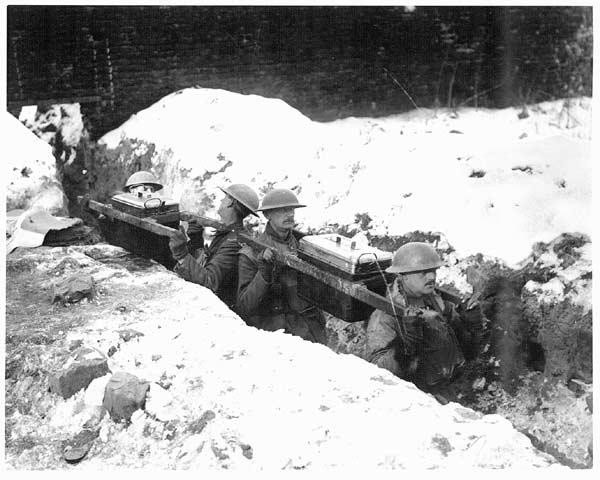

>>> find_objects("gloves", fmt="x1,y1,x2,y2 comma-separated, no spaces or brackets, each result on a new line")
169,230,187,259
187,220,206,248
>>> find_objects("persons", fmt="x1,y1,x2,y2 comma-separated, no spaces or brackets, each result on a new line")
234,188,329,347
365,240,487,411
120,170,164,200
165,182,261,311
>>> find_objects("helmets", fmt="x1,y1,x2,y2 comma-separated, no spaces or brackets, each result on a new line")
255,190,307,211
385,242,445,274
218,183,262,218
123,170,163,191
